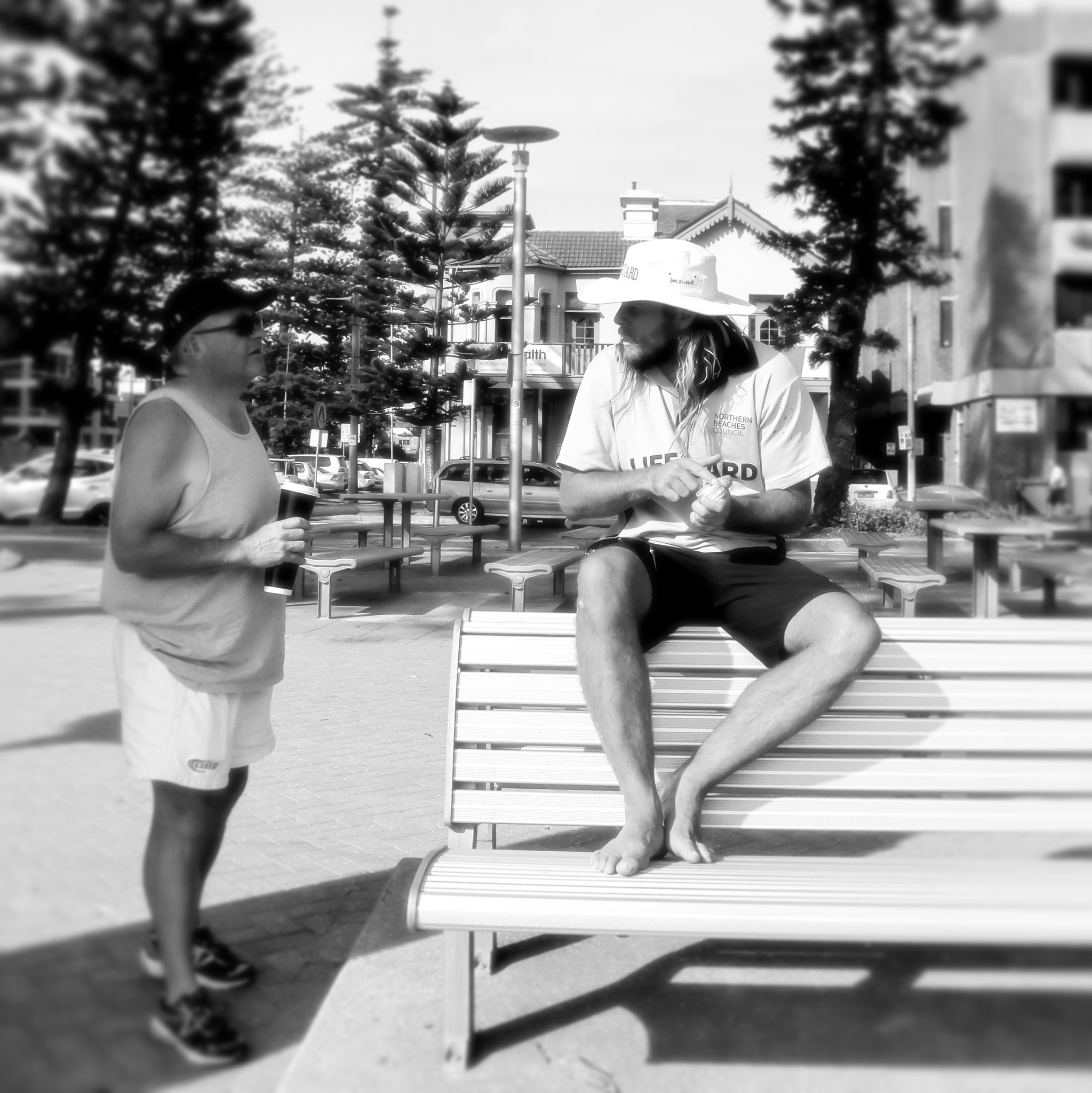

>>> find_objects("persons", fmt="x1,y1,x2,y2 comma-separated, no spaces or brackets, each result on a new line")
1046,463,1068,524
102,270,317,1072
554,237,881,882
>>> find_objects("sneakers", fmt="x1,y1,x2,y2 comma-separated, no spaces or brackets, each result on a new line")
140,923,261,994
144,982,249,1069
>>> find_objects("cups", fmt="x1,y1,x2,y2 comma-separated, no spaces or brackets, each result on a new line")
264,479,324,596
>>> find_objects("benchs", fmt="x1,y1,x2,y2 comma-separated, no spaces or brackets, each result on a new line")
558,524,613,544
402,604,1092,1074
482,547,587,612
294,518,502,620
837,525,948,615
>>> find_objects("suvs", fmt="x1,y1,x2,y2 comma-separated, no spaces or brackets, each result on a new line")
424,456,567,525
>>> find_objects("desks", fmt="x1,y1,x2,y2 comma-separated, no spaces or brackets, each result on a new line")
893,497,1055,617
338,491,452,568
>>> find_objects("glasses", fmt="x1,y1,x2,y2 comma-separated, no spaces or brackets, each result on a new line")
183,311,261,353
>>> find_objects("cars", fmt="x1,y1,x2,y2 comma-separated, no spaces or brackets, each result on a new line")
842,470,905,527
0,449,118,527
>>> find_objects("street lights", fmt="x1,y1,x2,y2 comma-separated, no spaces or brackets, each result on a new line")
327,284,370,494
483,125,559,553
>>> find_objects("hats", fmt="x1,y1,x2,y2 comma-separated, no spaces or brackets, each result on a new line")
577,237,758,317
163,276,279,346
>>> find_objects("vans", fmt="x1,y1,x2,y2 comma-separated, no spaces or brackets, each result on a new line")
268,452,390,493
